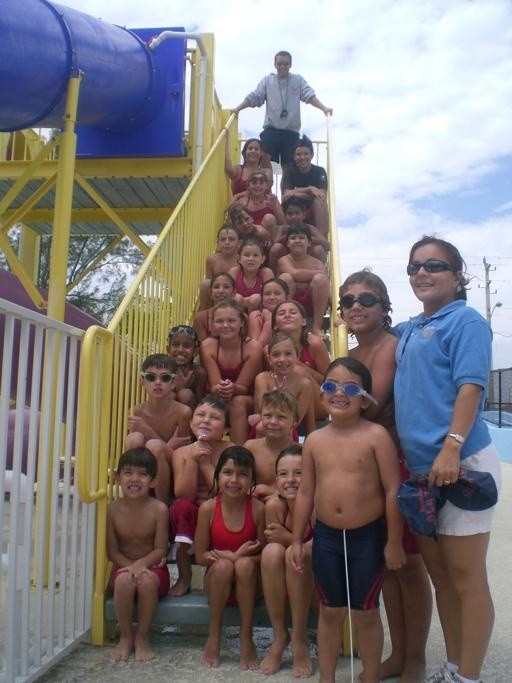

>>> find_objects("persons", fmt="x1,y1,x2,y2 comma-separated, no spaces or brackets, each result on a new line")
106,446,170,662
229,51,333,174
167,395,236,597
334,235,500,683
194,445,265,670
259,445,319,678
241,388,303,503
337,266,432,683
126,353,193,504
289,357,408,682
165,126,332,445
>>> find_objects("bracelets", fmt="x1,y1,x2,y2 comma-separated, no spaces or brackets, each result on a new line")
447,433,465,444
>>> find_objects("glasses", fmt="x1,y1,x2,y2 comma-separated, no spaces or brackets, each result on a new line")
248,176,266,181
320,381,379,406
167,327,198,349
276,62,288,65
407,259,456,276
141,372,176,384
341,295,384,308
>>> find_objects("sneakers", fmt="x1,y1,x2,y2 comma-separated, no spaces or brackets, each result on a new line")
440,671,484,683
424,660,459,682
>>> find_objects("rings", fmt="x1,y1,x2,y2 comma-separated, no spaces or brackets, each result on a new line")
445,480,450,485
